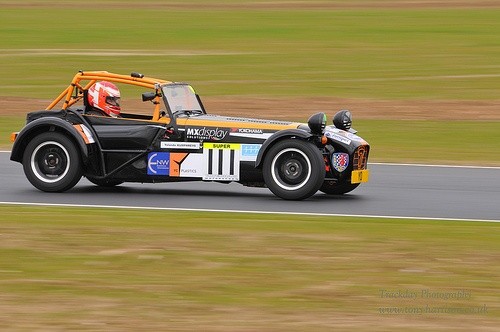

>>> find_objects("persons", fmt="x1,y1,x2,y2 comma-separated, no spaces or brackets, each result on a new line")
84,81,121,117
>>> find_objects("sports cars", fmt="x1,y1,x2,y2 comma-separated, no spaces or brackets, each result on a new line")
9,72,370,201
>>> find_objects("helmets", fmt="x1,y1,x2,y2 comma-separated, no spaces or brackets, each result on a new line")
87,81,121,117
